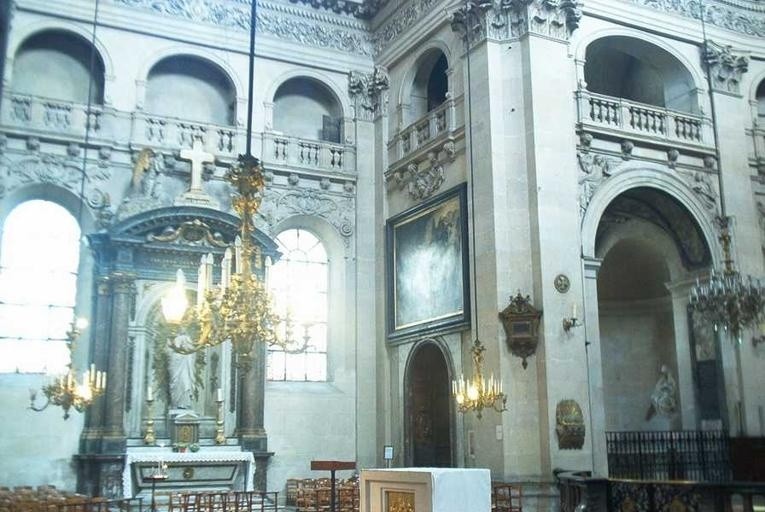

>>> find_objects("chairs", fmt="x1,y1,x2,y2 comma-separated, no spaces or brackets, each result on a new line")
285,478,360,512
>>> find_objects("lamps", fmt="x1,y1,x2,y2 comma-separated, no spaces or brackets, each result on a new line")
685,0,763,348
28,0,108,423
157,0,316,358
451,1,509,423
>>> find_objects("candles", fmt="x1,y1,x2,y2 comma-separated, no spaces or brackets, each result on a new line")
573,303,577,317
147,386,152,399
217,387,222,400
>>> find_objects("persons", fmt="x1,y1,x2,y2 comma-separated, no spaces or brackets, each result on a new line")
167,324,198,409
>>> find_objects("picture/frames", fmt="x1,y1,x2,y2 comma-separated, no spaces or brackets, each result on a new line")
385,180,471,347
